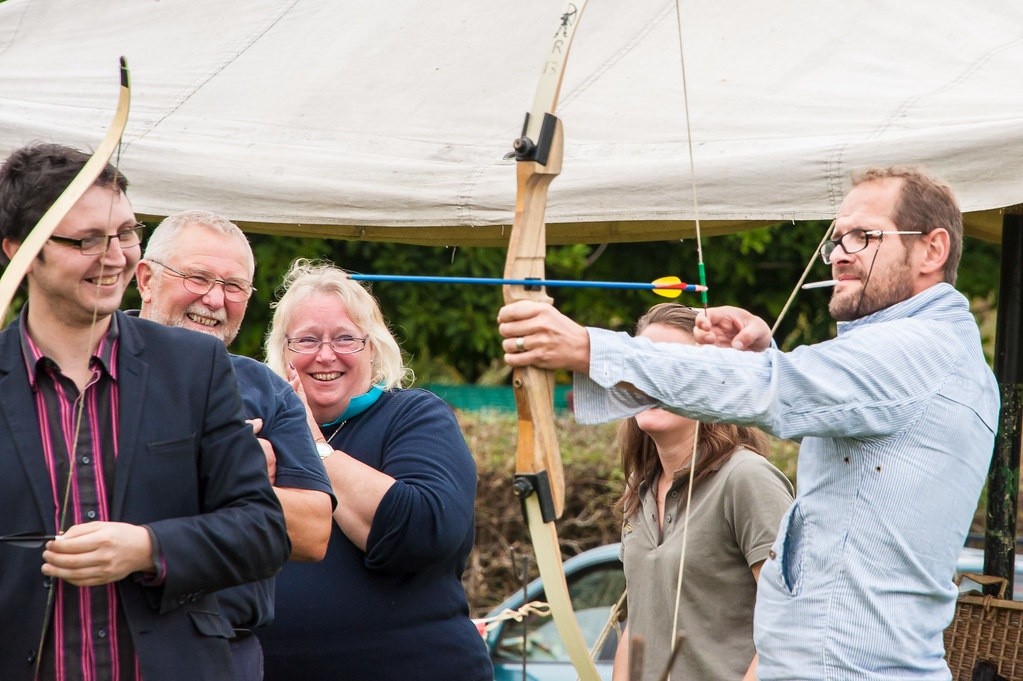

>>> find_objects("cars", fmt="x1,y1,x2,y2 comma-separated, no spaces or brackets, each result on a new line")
467,542,1023,681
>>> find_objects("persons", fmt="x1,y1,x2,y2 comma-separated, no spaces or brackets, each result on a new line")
611,303,795,680
498,166,1000,681
252,259,495,681
0,140,291,681
123,209,338,680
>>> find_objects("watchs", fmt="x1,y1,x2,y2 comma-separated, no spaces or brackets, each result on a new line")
315,442,334,459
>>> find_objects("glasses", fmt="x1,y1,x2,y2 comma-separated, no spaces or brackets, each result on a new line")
49,222,146,255
148,260,258,302
819,228,929,265
285,334,369,355
